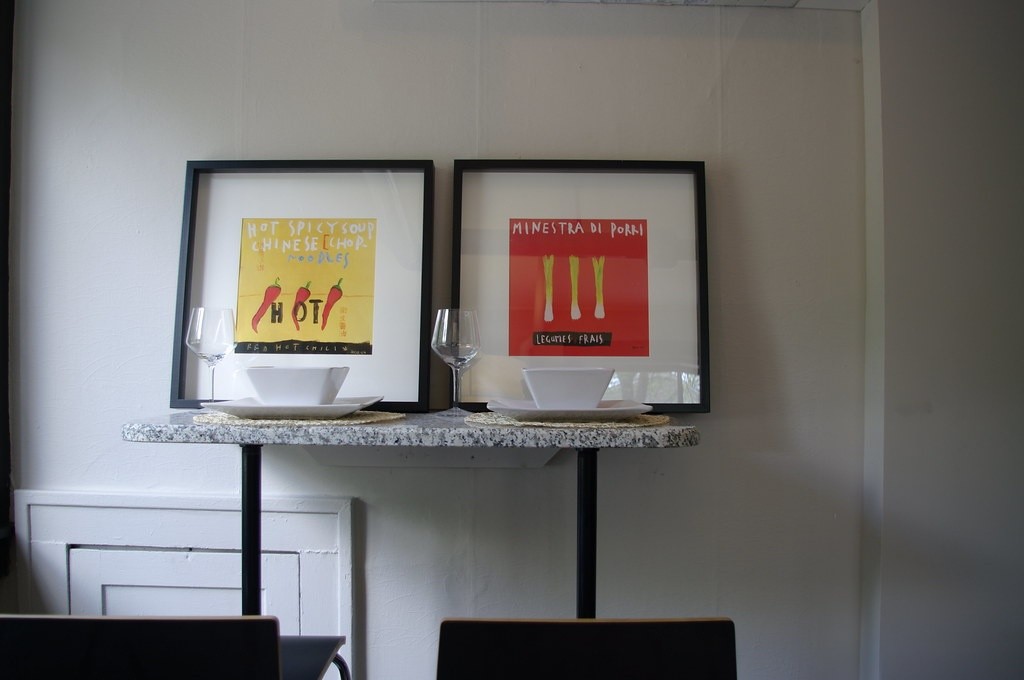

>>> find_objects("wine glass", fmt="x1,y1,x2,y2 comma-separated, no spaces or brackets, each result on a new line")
430,309,481,420
185,307,238,413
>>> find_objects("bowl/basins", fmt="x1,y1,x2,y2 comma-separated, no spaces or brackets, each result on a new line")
521,366,616,408
244,365,350,405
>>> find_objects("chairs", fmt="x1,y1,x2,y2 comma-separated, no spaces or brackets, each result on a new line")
436,619,739,680
0,613,352,680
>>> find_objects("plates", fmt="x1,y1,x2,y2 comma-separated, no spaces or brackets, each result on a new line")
199,396,385,420
487,399,653,423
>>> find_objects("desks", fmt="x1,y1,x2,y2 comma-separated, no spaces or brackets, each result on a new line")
124,409,700,618
